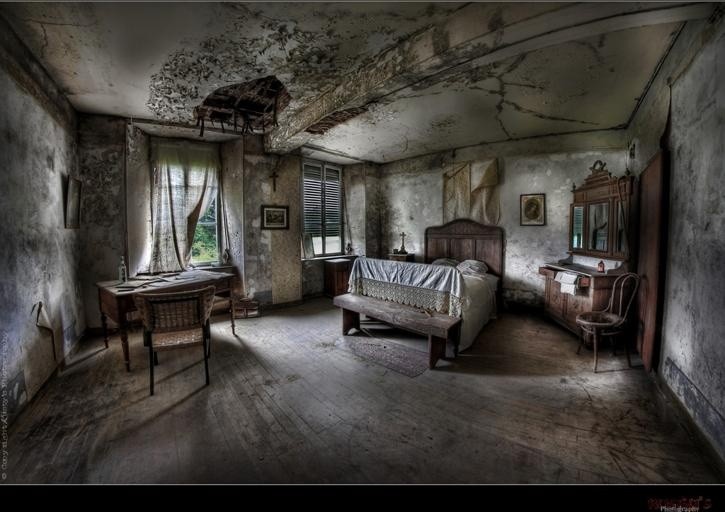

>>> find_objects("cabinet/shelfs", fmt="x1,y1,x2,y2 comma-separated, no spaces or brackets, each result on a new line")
326,259,352,299
539,262,631,343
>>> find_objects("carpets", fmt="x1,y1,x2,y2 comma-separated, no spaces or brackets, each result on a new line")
334,332,430,378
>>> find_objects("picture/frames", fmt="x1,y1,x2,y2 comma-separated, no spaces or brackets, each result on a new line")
519,193,546,226
260,204,290,230
64,175,83,229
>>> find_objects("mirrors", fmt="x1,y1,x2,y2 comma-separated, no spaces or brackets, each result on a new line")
568,197,626,253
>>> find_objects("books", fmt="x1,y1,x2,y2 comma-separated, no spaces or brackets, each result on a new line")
115,271,179,288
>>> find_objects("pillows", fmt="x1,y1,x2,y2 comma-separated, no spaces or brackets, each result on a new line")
431,257,488,276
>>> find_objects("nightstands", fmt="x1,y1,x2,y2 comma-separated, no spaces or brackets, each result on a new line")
388,253,414,262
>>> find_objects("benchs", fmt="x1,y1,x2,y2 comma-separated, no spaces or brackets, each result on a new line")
332,292,463,371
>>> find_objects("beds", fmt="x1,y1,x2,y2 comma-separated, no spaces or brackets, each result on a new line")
352,218,504,362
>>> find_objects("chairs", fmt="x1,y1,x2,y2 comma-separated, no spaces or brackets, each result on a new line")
133,286,216,397
574,271,641,375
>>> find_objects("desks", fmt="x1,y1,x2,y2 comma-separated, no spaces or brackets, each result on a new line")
94,269,237,374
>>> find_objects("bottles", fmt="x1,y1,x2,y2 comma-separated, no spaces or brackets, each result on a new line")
119,256,127,284
